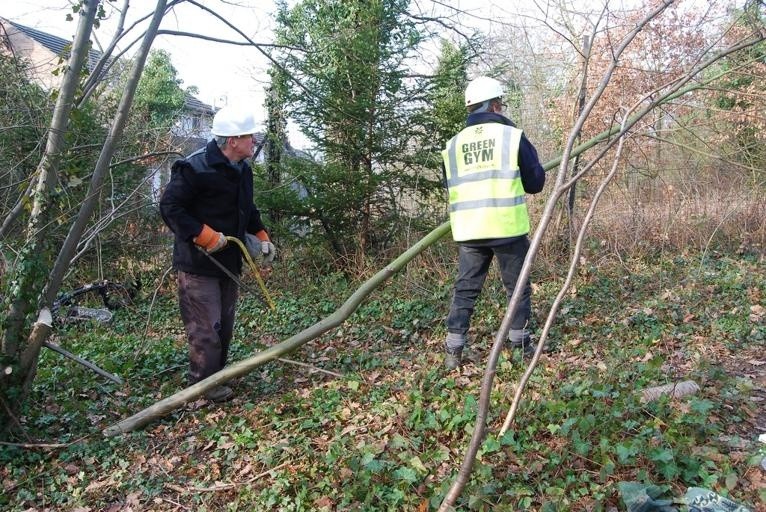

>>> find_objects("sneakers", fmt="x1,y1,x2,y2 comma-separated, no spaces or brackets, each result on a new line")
202,378,243,402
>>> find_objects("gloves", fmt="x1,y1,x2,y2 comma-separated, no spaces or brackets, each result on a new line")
254,229,274,267
193,224,226,255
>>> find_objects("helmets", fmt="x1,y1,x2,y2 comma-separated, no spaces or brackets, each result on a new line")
212,107,262,137
465,76,504,107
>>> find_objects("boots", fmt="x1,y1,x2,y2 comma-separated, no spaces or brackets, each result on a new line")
445,345,462,370
513,338,534,357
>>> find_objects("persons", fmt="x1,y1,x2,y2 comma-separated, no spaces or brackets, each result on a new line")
160,105,275,403
441,76,545,368
